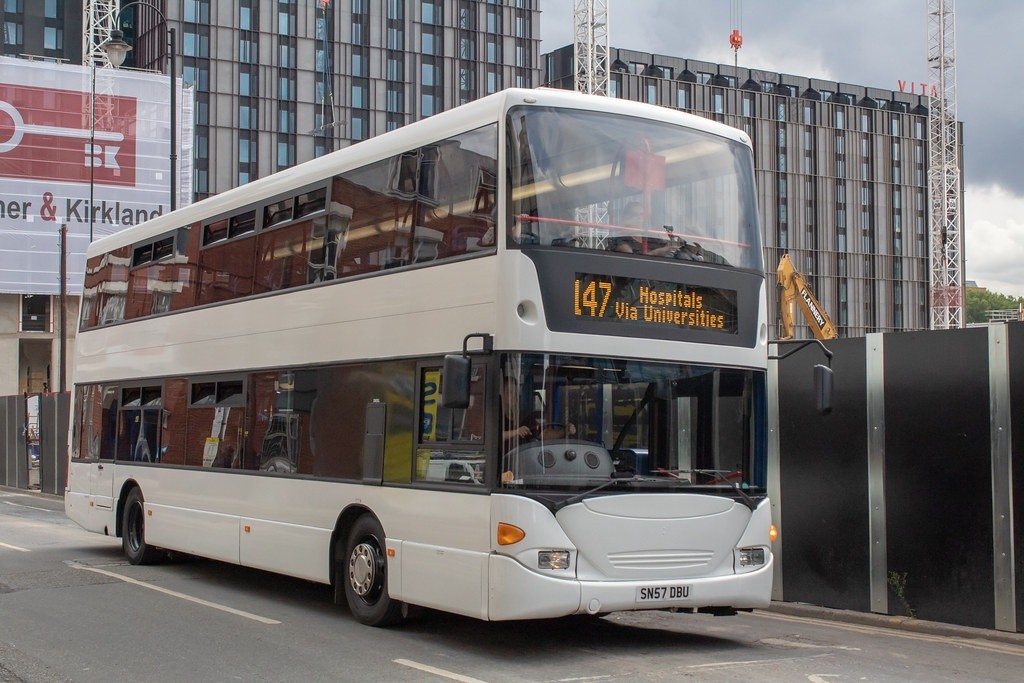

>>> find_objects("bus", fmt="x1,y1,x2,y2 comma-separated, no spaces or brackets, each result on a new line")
62,86,837,629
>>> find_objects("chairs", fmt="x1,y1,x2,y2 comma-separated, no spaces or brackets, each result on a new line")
450,224,536,252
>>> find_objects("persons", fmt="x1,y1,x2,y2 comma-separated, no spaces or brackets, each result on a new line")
615,202,704,261
482,208,530,244
474,376,577,451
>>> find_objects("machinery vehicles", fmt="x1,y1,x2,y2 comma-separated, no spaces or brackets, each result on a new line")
776,253,839,339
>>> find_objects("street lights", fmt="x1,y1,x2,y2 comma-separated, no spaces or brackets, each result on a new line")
100,1,177,211
